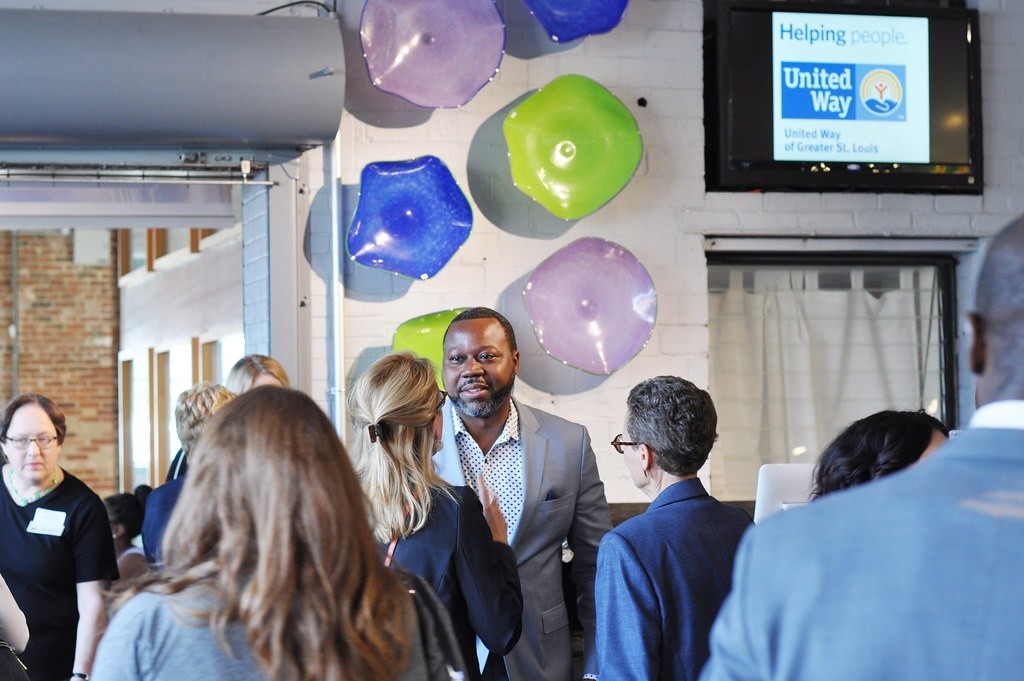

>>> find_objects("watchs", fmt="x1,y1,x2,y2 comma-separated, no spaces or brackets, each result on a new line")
70,672,91,681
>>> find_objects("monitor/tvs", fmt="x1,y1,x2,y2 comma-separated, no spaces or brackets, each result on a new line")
716,0,984,194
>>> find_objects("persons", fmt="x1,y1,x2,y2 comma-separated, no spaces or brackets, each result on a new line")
0,573,31,681
431,306,614,681
804,407,953,503
90,384,467,681
142,381,236,576
699,215,1024,681
0,394,122,681
104,485,154,586
583,377,756,681
347,352,524,681
164,353,288,487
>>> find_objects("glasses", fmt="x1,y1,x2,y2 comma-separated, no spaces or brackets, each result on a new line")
436,389,448,409
5,435,59,450
612,433,639,455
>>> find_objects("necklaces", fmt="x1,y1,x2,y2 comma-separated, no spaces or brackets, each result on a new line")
7,466,57,506
